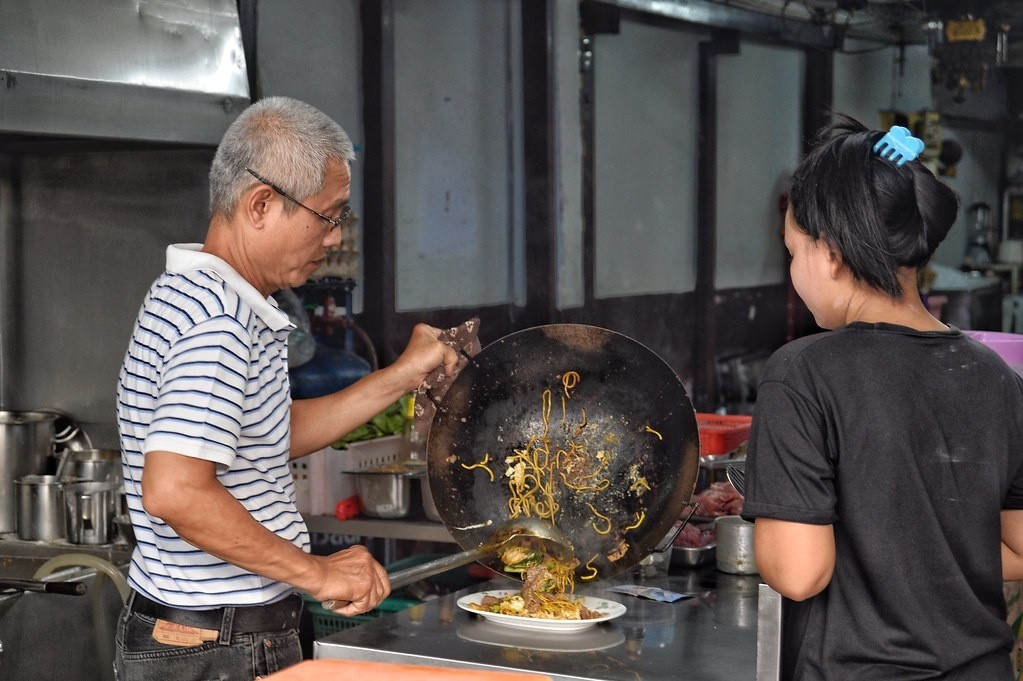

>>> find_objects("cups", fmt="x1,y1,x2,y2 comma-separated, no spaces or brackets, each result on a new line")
64,481,121,546
13,475,80,540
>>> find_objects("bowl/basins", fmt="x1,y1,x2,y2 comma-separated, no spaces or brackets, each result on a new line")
59,449,124,488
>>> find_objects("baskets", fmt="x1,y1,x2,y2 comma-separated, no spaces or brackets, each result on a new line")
307,553,485,640
289,435,402,517
696,412,753,458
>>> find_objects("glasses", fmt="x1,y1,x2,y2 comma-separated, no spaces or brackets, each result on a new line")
243,165,351,232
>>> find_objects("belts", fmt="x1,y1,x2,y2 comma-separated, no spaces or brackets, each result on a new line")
126,588,303,632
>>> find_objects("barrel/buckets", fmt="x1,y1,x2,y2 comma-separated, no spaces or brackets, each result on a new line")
0,409,63,535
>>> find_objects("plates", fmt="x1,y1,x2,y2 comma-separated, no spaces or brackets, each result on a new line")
457,589,627,630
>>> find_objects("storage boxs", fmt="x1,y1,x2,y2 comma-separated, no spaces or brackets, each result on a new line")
307,551,495,658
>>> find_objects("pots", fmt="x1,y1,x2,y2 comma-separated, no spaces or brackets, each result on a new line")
422,322,702,586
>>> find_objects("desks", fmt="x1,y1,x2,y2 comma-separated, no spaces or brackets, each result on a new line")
255,557,782,681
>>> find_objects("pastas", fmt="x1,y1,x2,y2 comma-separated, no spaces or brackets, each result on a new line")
462,370,663,621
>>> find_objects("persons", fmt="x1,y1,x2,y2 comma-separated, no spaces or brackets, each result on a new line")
740,112,1023,681
116,97,460,680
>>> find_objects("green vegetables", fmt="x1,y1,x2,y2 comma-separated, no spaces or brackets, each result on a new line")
330,393,415,450
